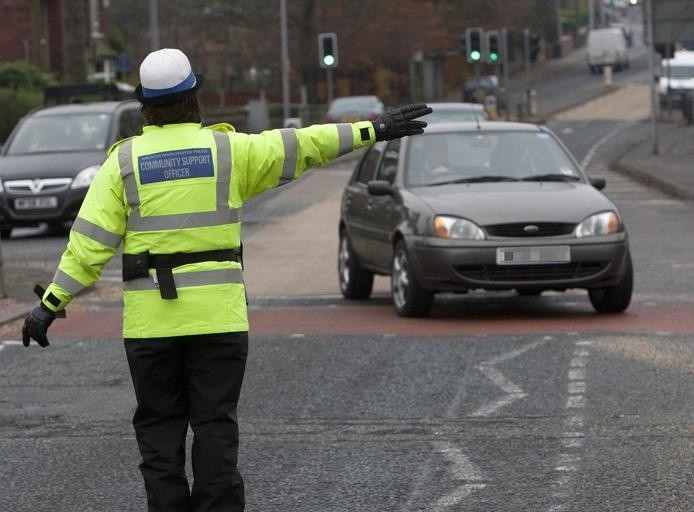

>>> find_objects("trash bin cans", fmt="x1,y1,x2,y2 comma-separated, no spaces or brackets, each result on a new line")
527,89,539,116
604,65,613,87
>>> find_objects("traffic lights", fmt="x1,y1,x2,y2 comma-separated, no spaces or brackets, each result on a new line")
461,28,540,65
319,32,339,69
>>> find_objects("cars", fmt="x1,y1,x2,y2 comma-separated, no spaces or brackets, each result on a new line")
324,93,384,125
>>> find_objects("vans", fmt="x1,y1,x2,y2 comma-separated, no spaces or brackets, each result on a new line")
586,26,628,73
0,100,147,238
654,57,694,109
465,75,507,111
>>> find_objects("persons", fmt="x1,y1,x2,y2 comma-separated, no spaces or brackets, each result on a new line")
22,48,434,512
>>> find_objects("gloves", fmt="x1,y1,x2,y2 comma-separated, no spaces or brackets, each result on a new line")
22,307,56,347
371,104,432,141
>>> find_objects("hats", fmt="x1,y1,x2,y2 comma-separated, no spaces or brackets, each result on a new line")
136,48,203,106
